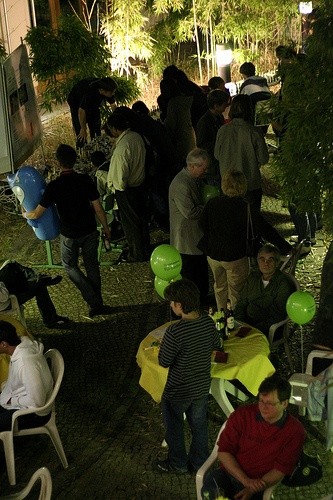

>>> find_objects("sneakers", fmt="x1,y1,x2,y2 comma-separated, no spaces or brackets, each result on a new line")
49,276,62,285
43,314,69,328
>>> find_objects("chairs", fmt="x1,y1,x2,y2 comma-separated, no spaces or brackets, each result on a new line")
196,420,283,500
268,238,333,417
98,193,124,267
0,295,68,500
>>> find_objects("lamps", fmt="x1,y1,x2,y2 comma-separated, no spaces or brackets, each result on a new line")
216,44,233,66
299,2,312,14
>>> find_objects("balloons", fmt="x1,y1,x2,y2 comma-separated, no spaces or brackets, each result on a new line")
286,291,317,325
149,243,182,280
154,274,184,301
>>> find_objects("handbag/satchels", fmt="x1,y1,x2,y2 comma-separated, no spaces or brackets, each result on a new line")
242,203,256,257
282,454,322,486
140,134,155,168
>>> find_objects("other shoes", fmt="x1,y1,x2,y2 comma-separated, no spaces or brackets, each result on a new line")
15,450,24,460
152,460,187,473
122,255,135,262
186,457,199,474
32,438,47,449
109,231,126,242
90,303,105,316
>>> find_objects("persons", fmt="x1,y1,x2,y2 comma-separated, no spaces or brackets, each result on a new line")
0,319,53,433
102,61,273,262
21,143,111,319
195,167,253,316
200,373,306,500
67,75,118,150
152,278,223,474
168,146,212,314
0,258,69,329
233,243,297,374
314,240,333,353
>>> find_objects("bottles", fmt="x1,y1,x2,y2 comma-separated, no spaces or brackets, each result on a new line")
214,299,236,341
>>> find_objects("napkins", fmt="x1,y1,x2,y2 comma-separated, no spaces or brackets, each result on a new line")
215,352,228,363
236,327,251,338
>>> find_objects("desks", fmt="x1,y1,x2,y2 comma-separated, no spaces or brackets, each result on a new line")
138,317,276,447
0,313,34,390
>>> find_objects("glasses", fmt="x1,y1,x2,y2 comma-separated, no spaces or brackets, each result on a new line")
259,398,280,406
